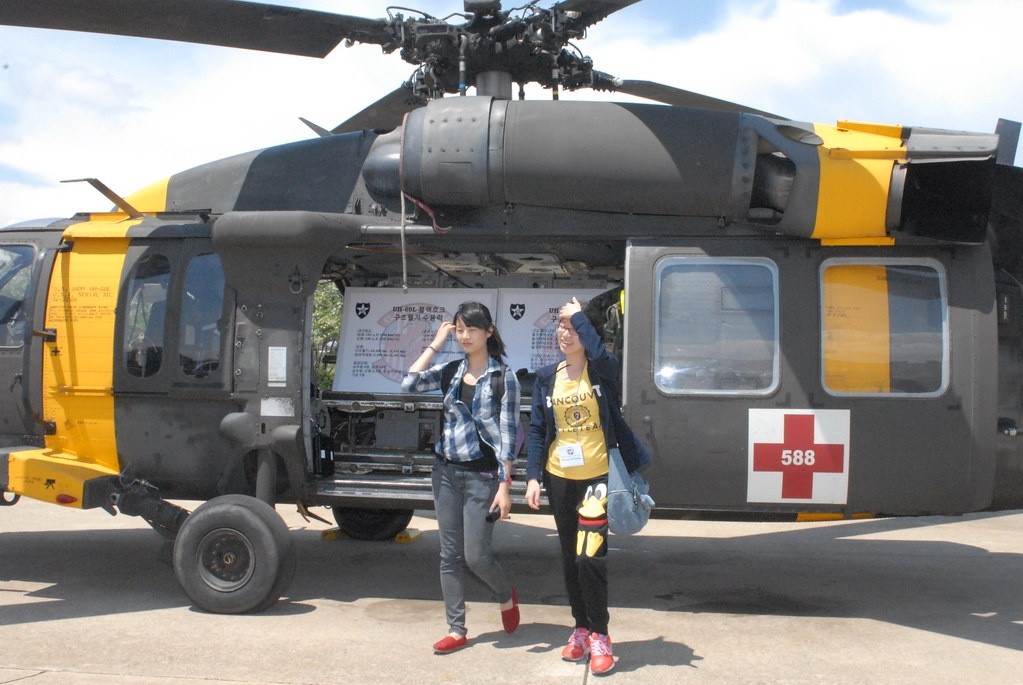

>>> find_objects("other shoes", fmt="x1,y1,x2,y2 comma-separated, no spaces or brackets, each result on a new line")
501,587,520,633
433,636,469,652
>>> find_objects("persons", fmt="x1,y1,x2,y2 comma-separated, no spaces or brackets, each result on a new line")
525,297,649,674
401,302,520,654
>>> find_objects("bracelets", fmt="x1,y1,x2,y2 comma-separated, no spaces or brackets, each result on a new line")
501,478,512,485
427,345,436,353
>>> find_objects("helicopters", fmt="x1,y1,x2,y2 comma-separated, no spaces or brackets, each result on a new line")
0,0,1022,616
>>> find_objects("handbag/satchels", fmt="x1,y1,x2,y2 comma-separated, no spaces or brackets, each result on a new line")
607,443,655,535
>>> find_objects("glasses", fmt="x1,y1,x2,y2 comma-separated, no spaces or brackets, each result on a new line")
554,324,578,336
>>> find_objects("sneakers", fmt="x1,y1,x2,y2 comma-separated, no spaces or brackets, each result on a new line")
589,633,614,674
561,627,592,661
